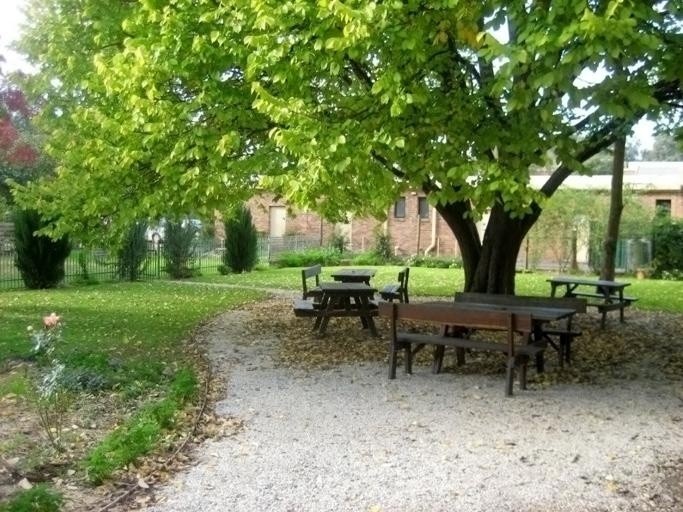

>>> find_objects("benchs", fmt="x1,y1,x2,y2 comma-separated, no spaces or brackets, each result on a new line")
378,292,586,397
558,292,639,328
294,264,410,333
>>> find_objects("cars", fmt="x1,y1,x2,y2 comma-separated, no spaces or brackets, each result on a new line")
144,217,203,244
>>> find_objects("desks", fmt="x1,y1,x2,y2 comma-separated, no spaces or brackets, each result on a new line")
547,277,631,328
